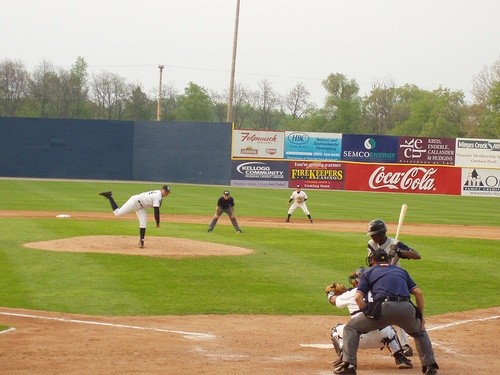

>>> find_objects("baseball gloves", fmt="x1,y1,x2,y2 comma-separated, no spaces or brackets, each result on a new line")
324,283,346,295
297,197,305,203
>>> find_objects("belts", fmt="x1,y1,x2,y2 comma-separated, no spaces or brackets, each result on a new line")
350,309,361,315
138,200,142,206
383,296,410,302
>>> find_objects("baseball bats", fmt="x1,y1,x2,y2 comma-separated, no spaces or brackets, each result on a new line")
394,204,408,244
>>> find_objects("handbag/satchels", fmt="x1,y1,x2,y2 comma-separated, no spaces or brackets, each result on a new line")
363,298,382,319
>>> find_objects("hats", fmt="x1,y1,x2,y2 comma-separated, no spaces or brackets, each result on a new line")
223,190,230,196
371,249,387,262
296,184,300,188
162,185,170,191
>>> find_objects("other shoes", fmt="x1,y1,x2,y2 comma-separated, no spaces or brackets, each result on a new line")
99,190,112,197
139,239,144,247
286,219,290,222
236,230,241,233
309,219,312,223
208,229,212,232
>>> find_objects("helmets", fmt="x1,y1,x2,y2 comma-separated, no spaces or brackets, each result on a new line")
367,219,385,236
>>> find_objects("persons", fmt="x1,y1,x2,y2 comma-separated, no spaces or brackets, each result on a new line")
324,219,440,375
208,190,242,233
285,185,313,223
98,185,170,248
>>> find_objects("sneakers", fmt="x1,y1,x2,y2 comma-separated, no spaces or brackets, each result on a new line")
334,362,356,375
333,358,342,366
395,356,413,369
402,344,413,356
421,361,440,375
